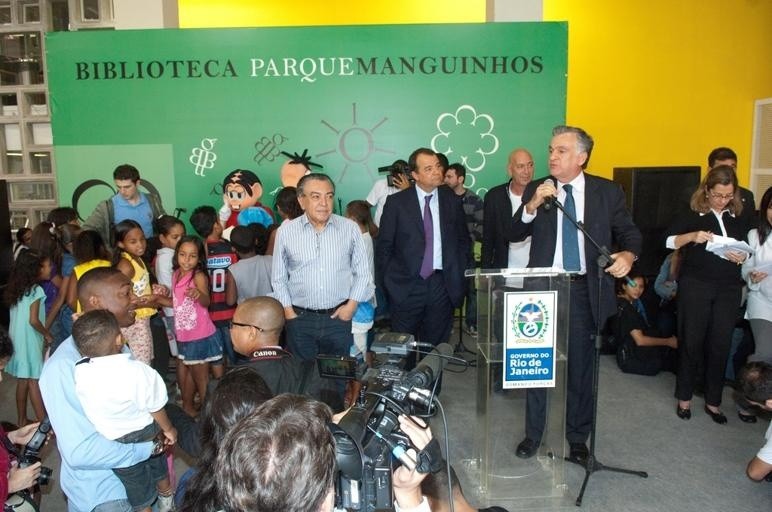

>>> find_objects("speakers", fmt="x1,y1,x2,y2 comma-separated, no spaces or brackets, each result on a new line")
613,166,700,330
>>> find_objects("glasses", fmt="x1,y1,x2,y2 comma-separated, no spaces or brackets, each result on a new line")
228,321,269,334
710,190,736,201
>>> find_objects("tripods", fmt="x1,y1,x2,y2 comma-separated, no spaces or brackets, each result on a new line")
451,303,477,355
548,259,648,506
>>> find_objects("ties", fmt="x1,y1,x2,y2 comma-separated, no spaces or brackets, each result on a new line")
562,184,581,271
419,195,434,282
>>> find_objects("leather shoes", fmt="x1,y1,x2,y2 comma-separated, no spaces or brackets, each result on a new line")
569,442,589,463
677,403,691,420
705,405,727,425
516,438,544,457
738,411,757,423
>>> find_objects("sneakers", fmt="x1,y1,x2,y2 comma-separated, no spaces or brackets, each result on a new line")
157,494,176,512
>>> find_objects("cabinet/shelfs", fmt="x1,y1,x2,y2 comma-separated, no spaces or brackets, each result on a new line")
748,98,770,211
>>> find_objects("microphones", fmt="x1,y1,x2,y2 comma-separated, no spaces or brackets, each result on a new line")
544,179,554,210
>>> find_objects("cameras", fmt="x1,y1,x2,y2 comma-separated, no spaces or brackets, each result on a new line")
18,413,54,485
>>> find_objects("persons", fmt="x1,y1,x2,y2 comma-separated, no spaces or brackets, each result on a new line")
481,125,643,461
0,148,507,512
613,147,771,483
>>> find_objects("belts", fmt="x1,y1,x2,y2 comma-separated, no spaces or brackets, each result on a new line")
293,300,349,315
